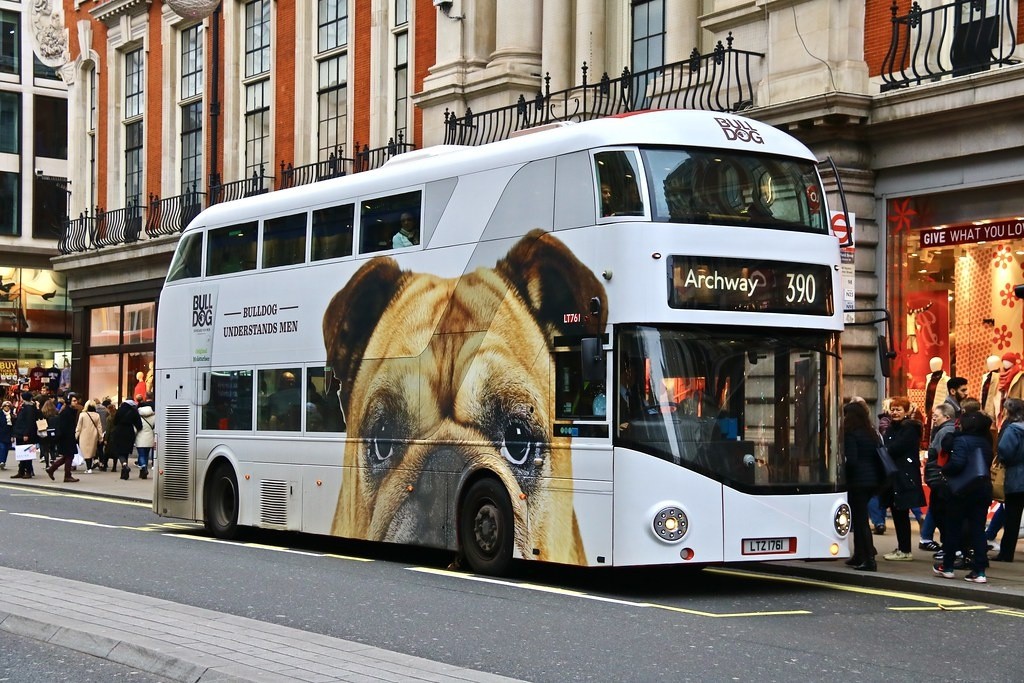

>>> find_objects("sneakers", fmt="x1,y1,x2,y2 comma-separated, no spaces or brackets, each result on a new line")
883,549,913,561
138,466,149,480
83,469,92,473
119,466,131,481
918,537,1015,583
844,555,877,572
871,525,887,534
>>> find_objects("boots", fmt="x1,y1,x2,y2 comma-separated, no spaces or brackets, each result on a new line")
9,463,32,479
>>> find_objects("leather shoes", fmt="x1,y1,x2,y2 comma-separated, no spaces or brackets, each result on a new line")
45,469,57,481
63,474,80,483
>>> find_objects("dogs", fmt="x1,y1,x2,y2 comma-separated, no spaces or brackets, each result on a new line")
321,228,609,565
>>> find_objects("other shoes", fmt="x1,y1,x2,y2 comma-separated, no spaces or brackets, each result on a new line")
0,462,7,471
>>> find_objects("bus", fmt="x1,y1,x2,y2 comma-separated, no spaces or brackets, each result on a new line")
152,108,855,578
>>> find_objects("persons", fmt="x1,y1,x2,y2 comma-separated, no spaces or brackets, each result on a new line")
988,396,1024,563
0,376,155,483
840,378,1012,583
923,352,1024,456
134,362,154,403
209,180,637,438
0,267,56,329
